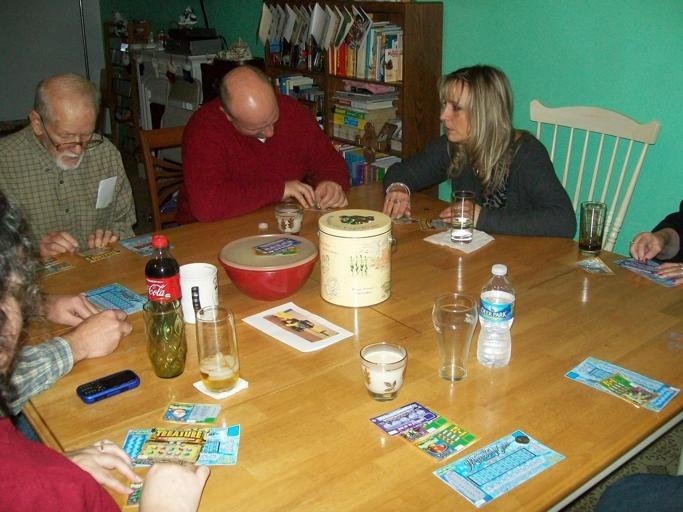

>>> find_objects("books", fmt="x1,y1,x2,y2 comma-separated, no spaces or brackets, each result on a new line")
251,0,404,187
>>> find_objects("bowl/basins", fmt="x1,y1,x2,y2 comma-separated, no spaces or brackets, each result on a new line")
216,234,319,302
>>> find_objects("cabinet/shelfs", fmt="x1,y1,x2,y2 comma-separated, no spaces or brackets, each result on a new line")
264,1,444,199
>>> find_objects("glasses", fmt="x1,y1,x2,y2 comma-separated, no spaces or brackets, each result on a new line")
37,112,103,152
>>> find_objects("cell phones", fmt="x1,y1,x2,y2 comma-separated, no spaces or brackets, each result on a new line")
77,369,140,403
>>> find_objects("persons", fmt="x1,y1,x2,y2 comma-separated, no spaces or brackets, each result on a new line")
173,63,350,227
381,62,578,240
628,198,683,288
0,71,139,262
0,291,134,420
0,187,211,511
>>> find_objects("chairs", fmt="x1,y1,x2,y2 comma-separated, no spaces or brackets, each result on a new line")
135,123,191,233
528,97,660,253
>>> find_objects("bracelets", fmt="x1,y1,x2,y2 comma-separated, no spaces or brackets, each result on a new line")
385,182,410,195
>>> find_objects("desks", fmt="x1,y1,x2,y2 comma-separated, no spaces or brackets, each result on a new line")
18,181,683,510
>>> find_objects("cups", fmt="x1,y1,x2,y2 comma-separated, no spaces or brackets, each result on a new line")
450,189,476,244
431,293,479,382
274,204,304,235
194,307,241,395
577,201,606,254
360,340,411,403
142,297,187,378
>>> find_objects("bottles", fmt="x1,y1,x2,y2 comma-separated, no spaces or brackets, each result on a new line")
143,234,181,310
475,264,517,370
175,262,220,326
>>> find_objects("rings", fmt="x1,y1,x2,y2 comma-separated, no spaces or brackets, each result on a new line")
678,262,683,267
678,265,683,270
392,197,400,204
99,444,105,455
678,270,683,277
388,197,394,202
629,239,638,246
399,199,409,206
